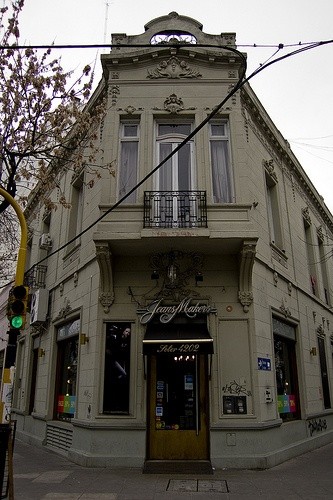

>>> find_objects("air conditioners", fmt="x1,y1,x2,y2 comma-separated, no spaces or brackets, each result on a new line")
29,288,49,326
39,233,53,251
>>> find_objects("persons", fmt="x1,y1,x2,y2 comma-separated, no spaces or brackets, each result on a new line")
104,326,132,382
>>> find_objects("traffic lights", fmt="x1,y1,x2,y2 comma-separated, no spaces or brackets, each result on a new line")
9,284,29,330
